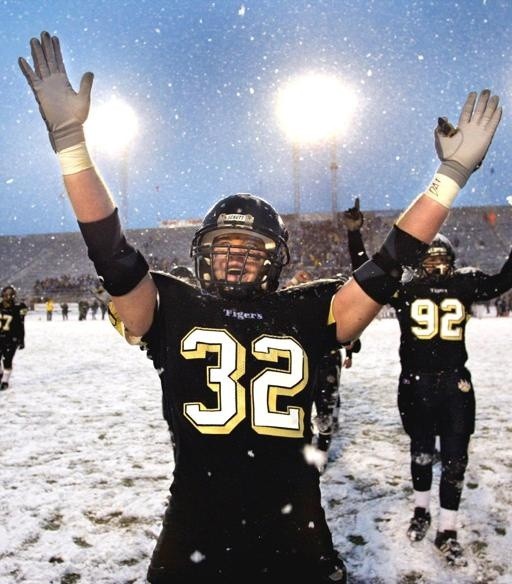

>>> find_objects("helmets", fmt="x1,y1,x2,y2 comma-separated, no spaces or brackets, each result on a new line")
415,231,457,284
1,284,17,302
189,193,292,301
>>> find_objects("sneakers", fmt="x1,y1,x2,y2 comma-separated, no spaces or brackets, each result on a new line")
0,381,8,391
406,505,433,542
430,528,470,570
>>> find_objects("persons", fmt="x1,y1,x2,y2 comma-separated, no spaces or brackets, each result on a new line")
47,297,108,321
280,272,361,473
495,289,512,317
342,198,512,570
17,30,503,583
0,286,28,391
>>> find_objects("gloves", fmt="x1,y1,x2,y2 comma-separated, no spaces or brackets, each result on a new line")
431,88,504,191
18,31,95,156
343,198,364,231
